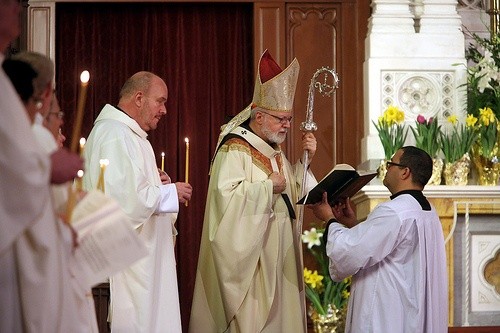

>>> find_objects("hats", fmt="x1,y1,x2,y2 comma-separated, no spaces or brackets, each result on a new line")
251,48,300,113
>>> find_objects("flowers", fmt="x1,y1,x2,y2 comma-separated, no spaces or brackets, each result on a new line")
371,3,500,167
296,223,352,316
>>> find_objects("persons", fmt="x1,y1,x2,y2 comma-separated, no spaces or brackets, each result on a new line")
0,0,86,333
306,145,449,333
188,48,318,333
74,70,193,333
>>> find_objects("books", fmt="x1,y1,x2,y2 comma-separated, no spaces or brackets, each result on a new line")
296,163,377,207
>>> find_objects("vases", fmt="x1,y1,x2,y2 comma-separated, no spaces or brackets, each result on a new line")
379,160,390,185
473,159,500,186
443,160,470,185
307,304,346,333
424,158,444,185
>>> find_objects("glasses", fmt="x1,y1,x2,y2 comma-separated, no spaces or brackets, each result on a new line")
32,95,46,110
51,111,64,119
258,110,294,124
385,161,404,170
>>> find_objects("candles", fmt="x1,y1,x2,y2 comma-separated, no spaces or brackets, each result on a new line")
67,69,190,206
